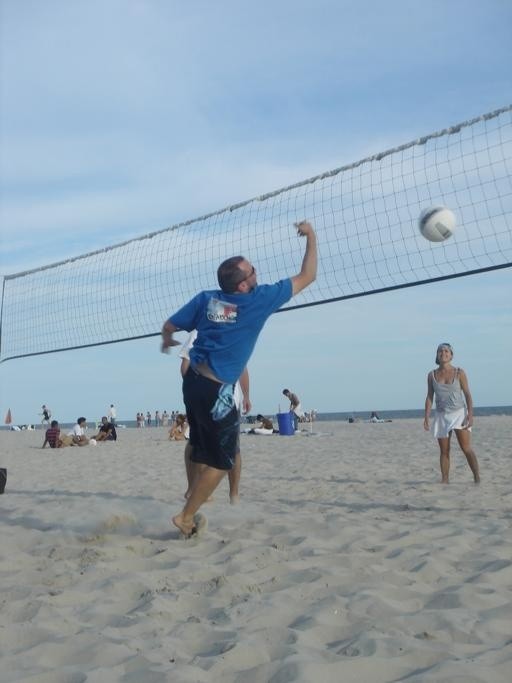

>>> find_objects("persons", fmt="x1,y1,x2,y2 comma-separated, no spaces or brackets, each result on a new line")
161,221,318,539
370,410,387,422
36,404,51,429
67,417,89,448
90,416,117,442
178,327,252,508
42,420,81,449
253,414,274,435
283,388,307,424
423,342,481,485
107,404,118,429
136,410,191,441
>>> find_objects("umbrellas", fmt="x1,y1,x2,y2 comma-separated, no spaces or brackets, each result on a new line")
4,408,14,424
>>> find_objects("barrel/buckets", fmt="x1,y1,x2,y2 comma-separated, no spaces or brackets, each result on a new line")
276,412,295,436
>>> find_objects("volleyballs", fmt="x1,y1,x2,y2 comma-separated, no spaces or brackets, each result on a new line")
419,206,454,241
